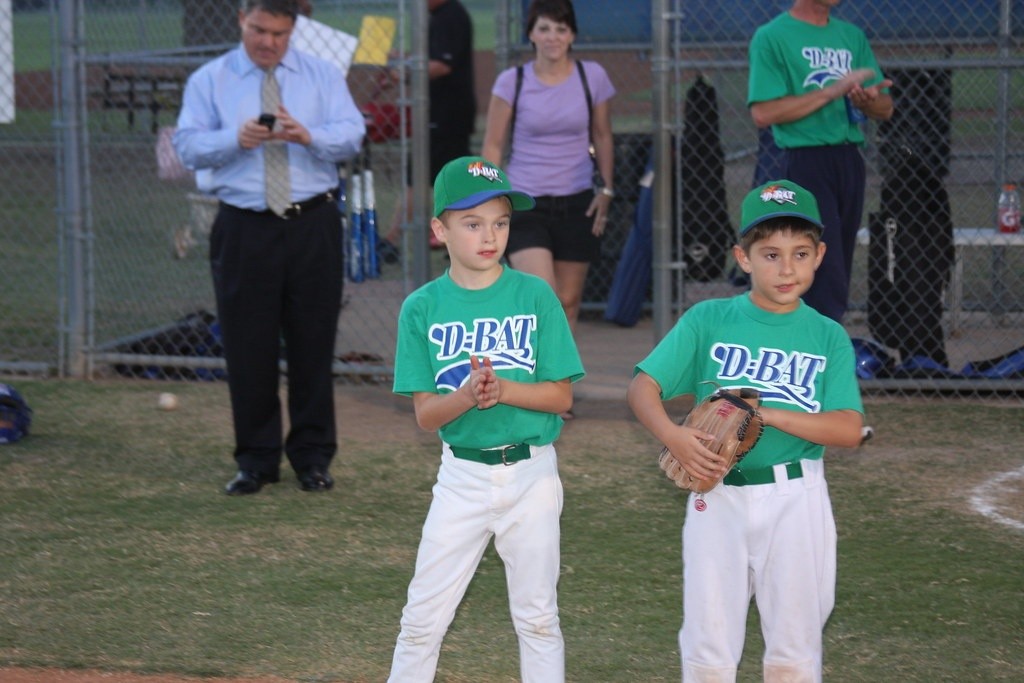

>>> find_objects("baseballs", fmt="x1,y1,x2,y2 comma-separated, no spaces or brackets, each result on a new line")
158,392,178,412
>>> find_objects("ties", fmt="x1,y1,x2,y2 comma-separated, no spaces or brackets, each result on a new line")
261,68,291,218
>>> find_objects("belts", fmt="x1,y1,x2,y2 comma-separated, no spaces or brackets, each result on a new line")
723,464,803,486
291,188,339,217
449,439,531,466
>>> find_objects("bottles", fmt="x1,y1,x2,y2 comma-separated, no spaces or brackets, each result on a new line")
997,184,1022,233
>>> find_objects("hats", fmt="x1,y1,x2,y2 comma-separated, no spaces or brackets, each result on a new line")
739,179,824,241
432,157,535,217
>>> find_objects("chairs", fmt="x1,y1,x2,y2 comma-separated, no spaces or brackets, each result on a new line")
100,64,185,132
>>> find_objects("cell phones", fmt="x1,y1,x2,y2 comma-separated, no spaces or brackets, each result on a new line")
259,113,276,132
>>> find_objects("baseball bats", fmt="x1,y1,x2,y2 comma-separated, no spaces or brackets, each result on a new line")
336,113,382,284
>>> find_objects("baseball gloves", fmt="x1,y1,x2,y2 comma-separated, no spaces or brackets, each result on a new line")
658,387,765,496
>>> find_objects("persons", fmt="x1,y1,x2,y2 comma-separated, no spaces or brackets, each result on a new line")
385,156,588,683
626,179,866,683
169,0,366,496
746,0,894,323
377,0,477,265
482,0,617,335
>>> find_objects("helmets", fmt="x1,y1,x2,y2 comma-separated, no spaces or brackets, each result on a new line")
0,384,31,444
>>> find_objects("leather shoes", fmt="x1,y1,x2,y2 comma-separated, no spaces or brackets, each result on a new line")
300,469,333,491
225,469,278,495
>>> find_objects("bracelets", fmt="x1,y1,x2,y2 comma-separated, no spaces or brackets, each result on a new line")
601,188,615,198
